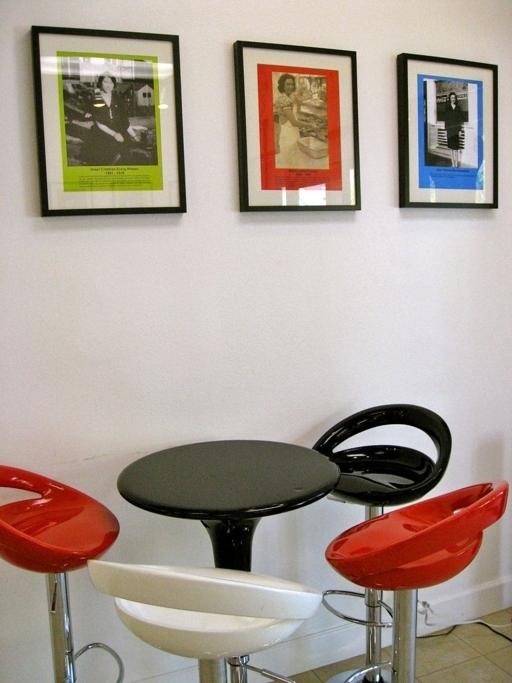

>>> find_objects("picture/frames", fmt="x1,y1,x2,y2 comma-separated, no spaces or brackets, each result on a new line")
396,52,499,207
232,39,362,212
30,23,188,217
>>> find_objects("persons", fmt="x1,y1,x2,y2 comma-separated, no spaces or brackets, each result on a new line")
273,74,320,155
445,92,464,168
79,71,141,165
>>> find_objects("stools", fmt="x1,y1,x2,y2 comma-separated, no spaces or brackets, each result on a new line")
1,462,128,683
85,552,323,683
325,477,511,682
307,403,453,682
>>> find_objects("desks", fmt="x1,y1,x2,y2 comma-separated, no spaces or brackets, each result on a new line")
116,438,344,683
